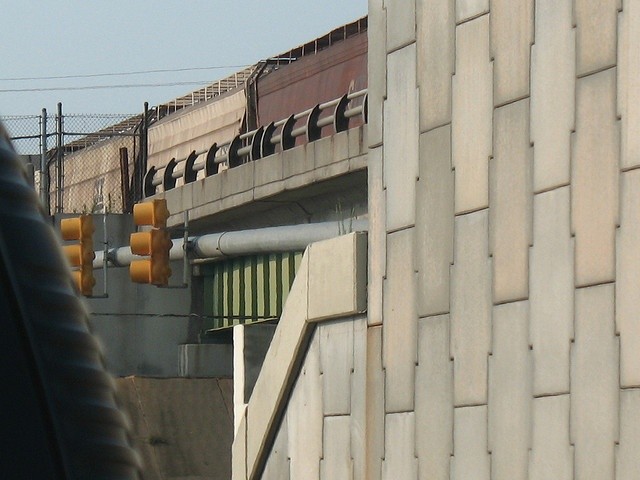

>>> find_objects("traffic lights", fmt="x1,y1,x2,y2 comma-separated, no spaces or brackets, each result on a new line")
60,214,94,295
129,199,171,286
